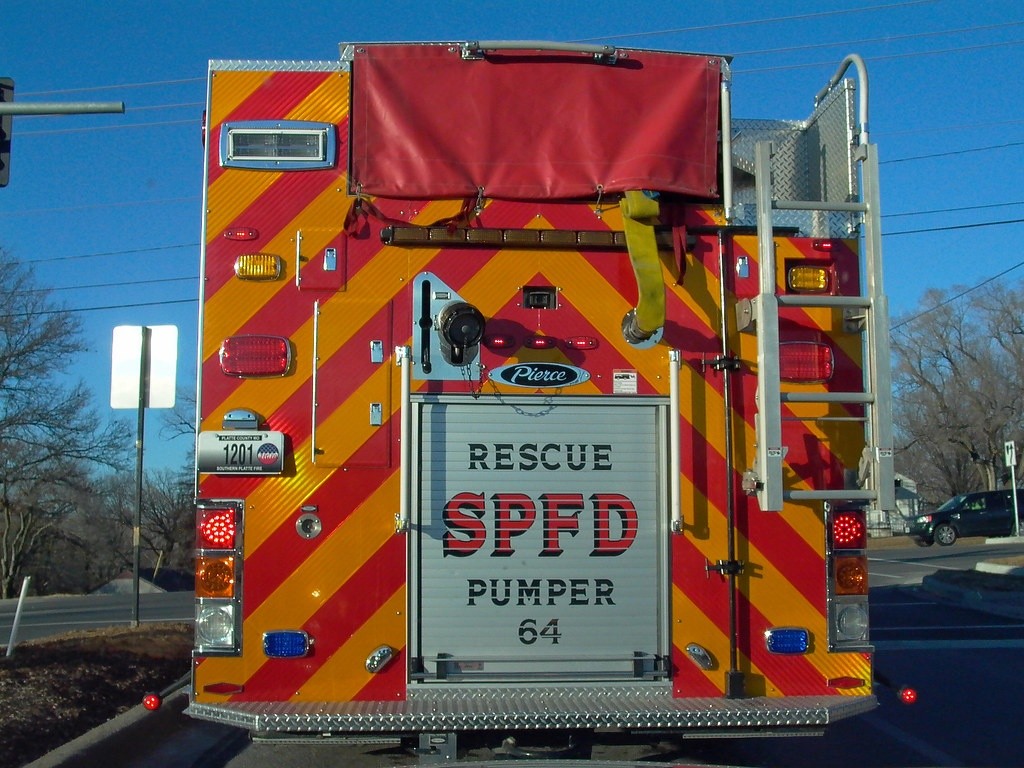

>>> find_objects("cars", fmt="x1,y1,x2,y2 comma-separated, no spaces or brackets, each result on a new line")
902,488,1024,547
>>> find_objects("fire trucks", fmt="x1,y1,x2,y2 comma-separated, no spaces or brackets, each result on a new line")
144,37,915,768
